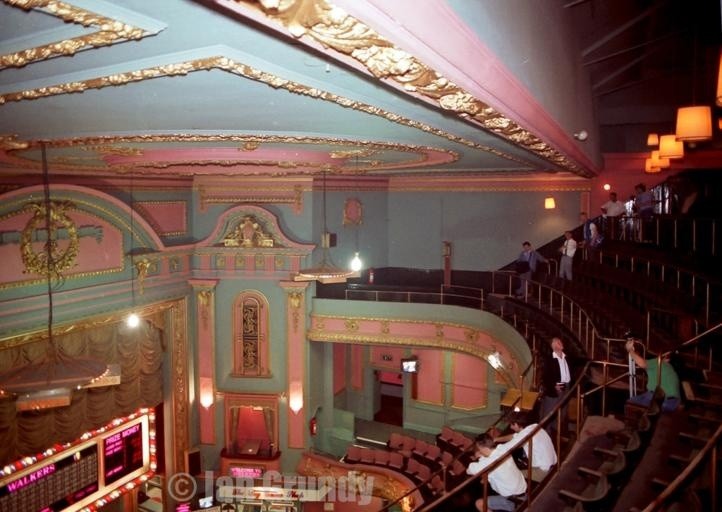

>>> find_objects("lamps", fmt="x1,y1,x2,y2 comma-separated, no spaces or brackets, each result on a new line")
125,165,140,330
199,376,215,413
349,150,367,273
643,49,722,174
288,377,303,418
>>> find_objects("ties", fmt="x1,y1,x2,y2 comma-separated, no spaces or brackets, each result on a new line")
564,239,569,256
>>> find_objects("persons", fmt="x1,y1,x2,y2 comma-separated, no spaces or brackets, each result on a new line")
541,336,578,453
600,191,628,240
633,184,643,239
624,337,681,411
558,231,577,281
466,434,529,511
577,212,599,260
492,410,557,484
513,240,545,296
633,182,655,244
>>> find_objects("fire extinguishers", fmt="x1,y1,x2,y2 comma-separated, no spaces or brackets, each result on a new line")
309,416,317,436
367,267,375,284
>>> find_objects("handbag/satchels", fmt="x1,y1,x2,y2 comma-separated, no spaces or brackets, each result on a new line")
515,259,530,274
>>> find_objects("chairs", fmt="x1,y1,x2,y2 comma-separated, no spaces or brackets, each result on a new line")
344,389,721,512
